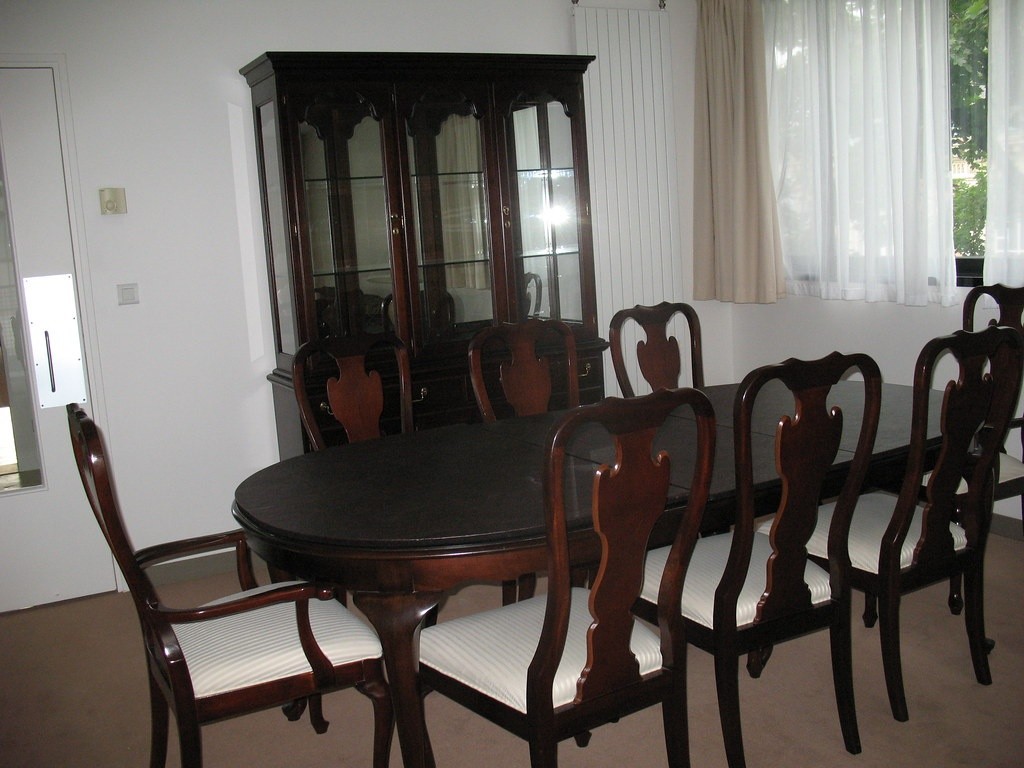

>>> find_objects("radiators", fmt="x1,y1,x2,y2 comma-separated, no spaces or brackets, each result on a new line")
571,1,688,399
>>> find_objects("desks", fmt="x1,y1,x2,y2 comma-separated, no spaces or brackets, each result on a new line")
232,381,963,768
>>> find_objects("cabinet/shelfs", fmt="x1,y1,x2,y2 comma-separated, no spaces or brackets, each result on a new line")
236,52,597,460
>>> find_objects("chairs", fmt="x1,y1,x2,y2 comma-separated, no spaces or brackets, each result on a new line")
609,301,703,399
313,273,544,334
901,281,1024,617
747,323,1022,724
466,318,580,603
416,384,719,767
584,350,882,765
290,331,439,628
63,403,395,768
